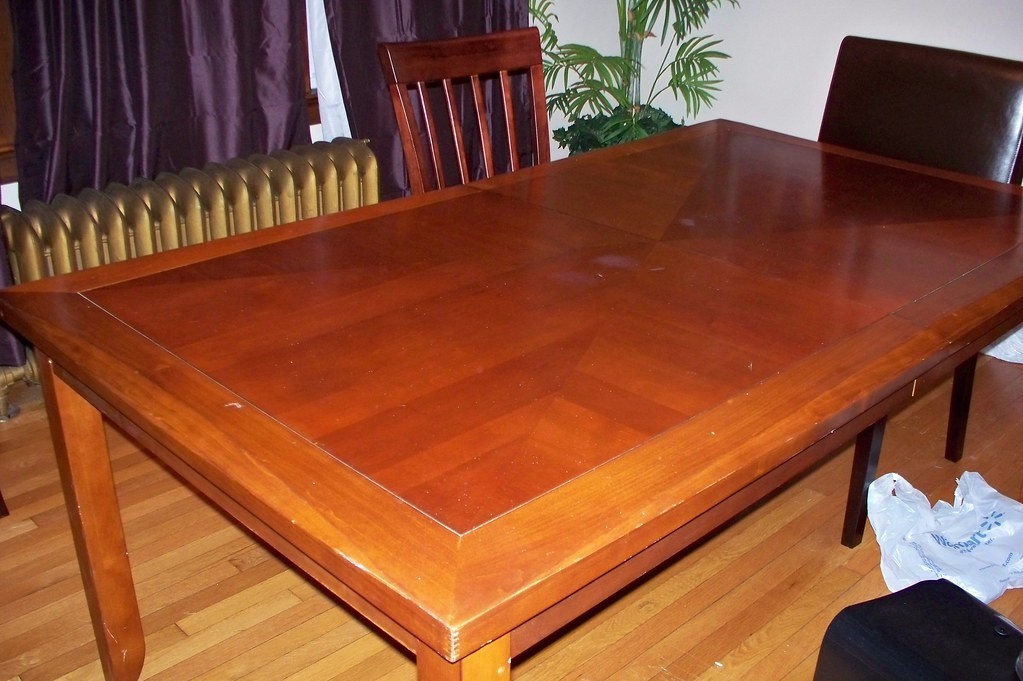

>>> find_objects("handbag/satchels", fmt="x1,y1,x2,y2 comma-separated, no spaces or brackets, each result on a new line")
868,472,1023,603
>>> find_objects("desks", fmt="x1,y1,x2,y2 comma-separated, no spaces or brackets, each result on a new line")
0,119,1023,681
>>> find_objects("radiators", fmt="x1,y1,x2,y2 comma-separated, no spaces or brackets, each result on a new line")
0,137,379,399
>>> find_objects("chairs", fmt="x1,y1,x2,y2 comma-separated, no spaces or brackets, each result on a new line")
807,35,1023,549
380,26,550,195
